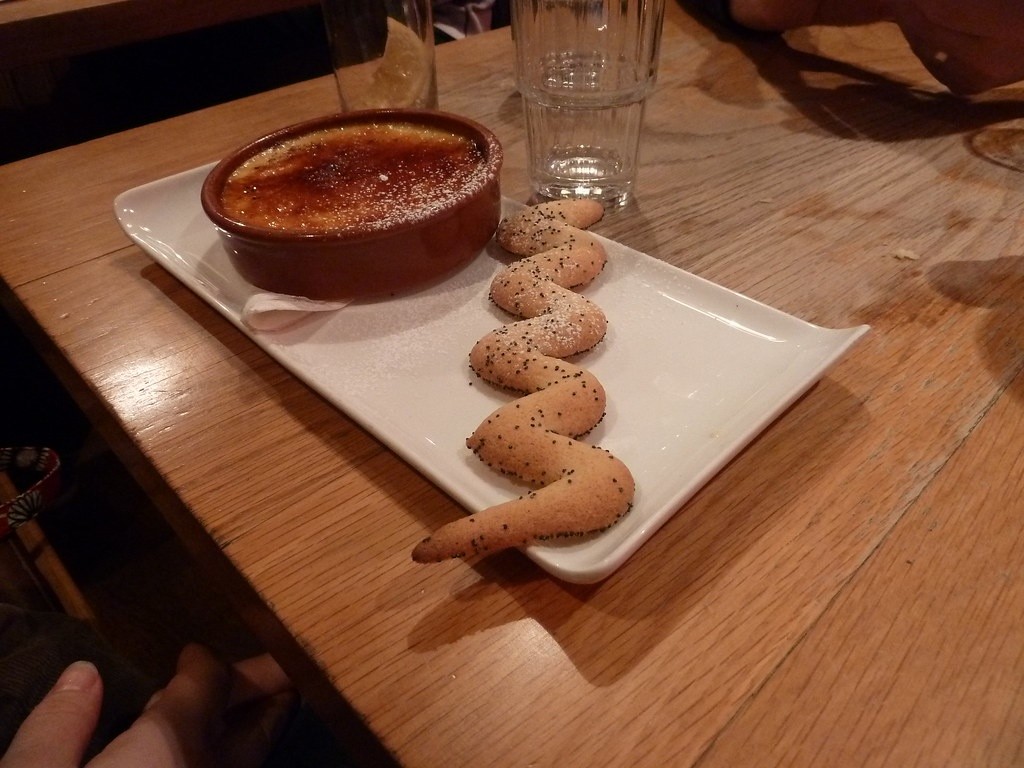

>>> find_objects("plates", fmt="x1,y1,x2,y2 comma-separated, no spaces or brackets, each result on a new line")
114,157,869,593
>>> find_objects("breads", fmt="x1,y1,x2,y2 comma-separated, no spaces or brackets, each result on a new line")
411,198,636,564
336,17,426,110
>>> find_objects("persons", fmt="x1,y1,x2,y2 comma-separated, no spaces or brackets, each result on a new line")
0,603,302,768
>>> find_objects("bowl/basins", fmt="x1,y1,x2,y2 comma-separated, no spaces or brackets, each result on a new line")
199,106,500,308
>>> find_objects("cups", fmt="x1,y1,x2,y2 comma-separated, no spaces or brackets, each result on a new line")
512,0,665,218
319,0,440,110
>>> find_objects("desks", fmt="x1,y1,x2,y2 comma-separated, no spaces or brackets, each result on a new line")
0,0,1024,768
